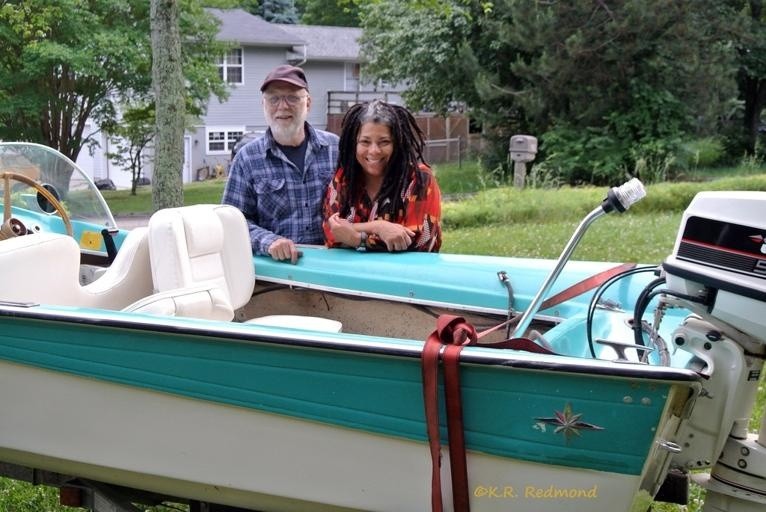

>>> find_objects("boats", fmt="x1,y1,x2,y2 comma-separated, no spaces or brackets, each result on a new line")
0,137,766,512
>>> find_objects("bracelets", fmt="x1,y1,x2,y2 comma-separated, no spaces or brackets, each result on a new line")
356,231,367,253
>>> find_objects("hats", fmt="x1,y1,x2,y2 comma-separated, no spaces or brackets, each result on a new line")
260,64,309,93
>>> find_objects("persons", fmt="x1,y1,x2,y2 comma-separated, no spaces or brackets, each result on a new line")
322,97,441,253
220,66,341,264
